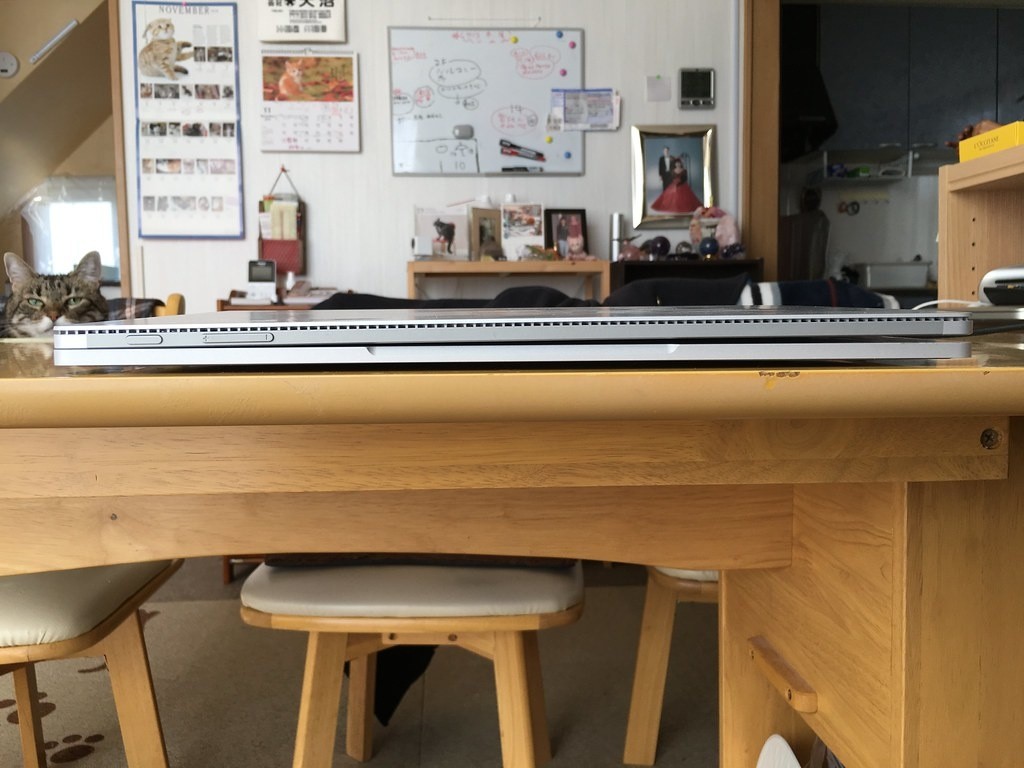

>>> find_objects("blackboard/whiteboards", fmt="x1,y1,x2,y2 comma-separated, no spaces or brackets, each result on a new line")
387,24,588,179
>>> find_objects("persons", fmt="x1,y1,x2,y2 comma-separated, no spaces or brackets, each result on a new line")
556,214,581,258
650,157,704,213
945,119,1001,159
659,147,675,192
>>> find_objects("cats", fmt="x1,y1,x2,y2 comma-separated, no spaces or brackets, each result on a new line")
0,251,110,349
277,59,324,101
433,218,455,254
136,5,239,212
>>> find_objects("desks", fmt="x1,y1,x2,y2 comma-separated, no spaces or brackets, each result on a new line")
0,146,1024,768
408,261,611,304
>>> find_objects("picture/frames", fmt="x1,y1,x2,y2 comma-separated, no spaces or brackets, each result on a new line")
471,206,501,257
545,209,589,259
631,124,720,230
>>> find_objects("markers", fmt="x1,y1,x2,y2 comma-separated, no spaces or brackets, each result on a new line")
501,166,544,174
498,138,547,162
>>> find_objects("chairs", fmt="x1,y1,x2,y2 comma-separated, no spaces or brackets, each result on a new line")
0,293,187,768
241,290,584,768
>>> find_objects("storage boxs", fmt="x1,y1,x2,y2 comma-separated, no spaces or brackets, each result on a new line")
958,119,1024,163
852,261,932,292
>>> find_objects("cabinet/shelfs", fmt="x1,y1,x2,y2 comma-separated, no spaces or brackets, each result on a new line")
611,258,767,294
792,4,1024,170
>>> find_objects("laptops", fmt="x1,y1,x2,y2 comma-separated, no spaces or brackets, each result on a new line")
52,306,975,367
